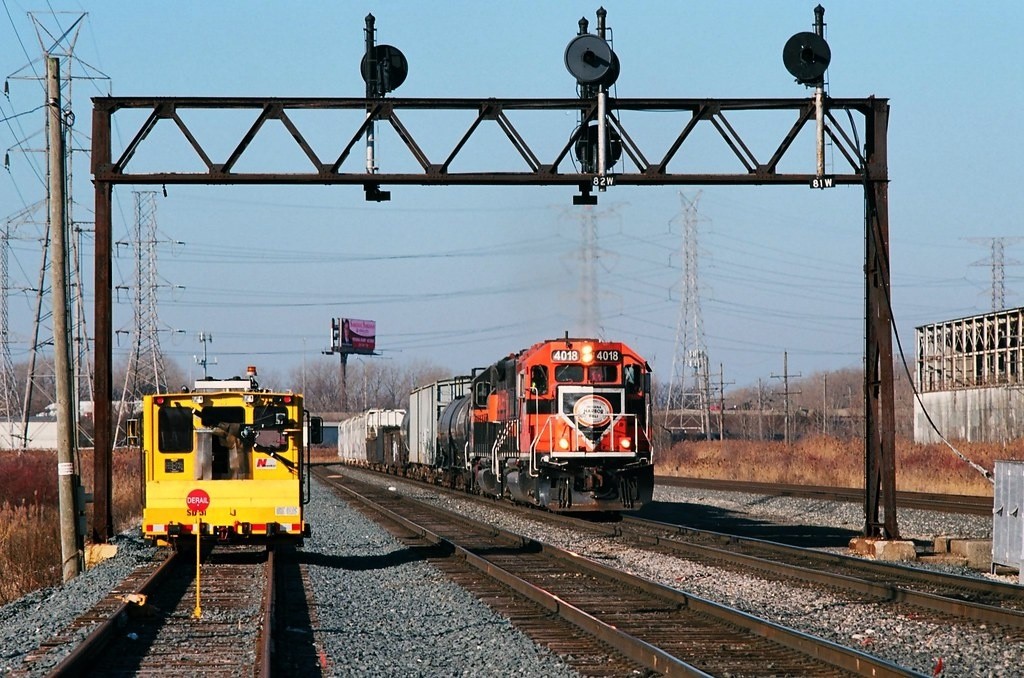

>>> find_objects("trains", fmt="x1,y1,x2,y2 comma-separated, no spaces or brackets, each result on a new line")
338,330,655,514
140,365,325,548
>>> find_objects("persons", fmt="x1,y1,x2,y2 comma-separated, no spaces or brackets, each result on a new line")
342,319,353,344
